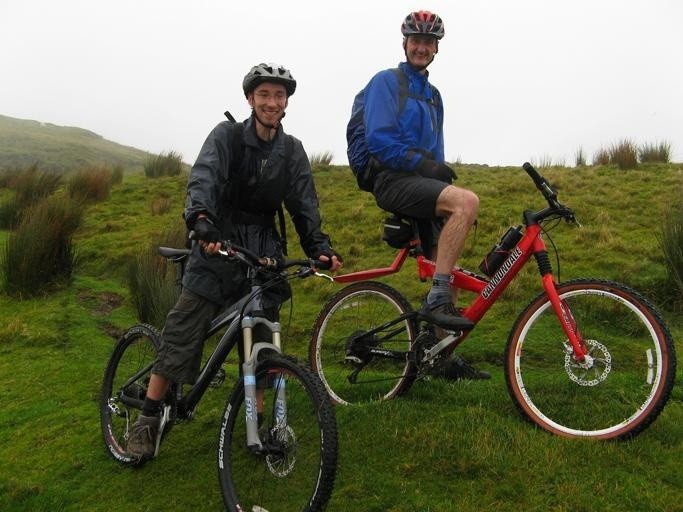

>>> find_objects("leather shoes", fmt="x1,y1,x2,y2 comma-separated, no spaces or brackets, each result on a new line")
417,291,474,329
431,356,490,379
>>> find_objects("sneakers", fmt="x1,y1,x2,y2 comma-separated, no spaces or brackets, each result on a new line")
128,415,159,456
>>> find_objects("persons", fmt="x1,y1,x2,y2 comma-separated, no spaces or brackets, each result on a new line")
346,9,492,380
127,63,344,460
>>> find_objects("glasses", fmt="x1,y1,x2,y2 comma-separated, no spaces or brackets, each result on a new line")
255,91,285,100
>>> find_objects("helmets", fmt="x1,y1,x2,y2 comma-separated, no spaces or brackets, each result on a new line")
243,63,297,100
401,10,445,39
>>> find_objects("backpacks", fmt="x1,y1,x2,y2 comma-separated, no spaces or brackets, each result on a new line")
346,69,441,192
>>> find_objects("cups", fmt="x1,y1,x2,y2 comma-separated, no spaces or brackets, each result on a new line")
478,227,528,278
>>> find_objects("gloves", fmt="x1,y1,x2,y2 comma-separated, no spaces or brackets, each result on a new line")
416,156,458,185
